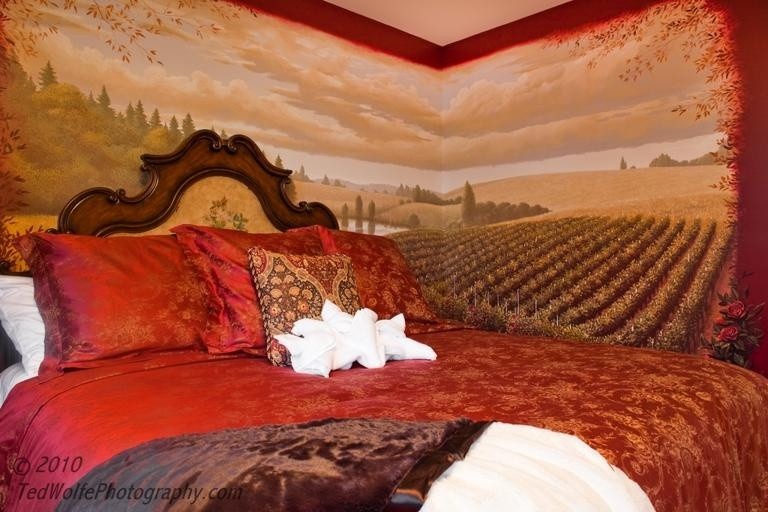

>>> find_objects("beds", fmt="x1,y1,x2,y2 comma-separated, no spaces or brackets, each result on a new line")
0,129,767,511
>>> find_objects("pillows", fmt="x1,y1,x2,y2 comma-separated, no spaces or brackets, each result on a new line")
287,223,448,335
1,274,45,380
12,231,210,384
247,246,362,367
169,224,335,359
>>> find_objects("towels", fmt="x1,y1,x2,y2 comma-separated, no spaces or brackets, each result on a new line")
271,298,438,380
